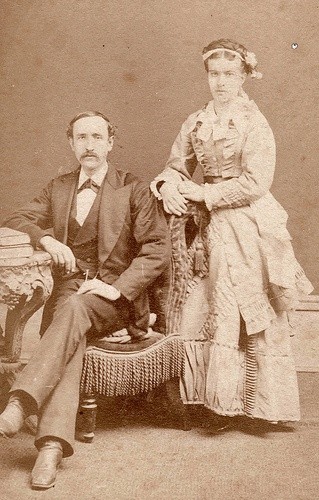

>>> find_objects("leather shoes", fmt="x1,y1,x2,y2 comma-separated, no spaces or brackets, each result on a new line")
30,441,63,488
0,395,30,439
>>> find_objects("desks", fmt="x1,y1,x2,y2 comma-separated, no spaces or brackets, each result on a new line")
0,254,54,400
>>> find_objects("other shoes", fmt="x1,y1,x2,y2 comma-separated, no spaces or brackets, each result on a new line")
204,413,238,435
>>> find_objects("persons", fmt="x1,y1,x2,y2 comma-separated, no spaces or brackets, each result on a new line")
148,38,314,435
1,111,176,490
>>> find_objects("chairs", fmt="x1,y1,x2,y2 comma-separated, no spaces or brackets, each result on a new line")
76,191,198,446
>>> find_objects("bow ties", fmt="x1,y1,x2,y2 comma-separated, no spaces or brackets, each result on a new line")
75,178,100,194
